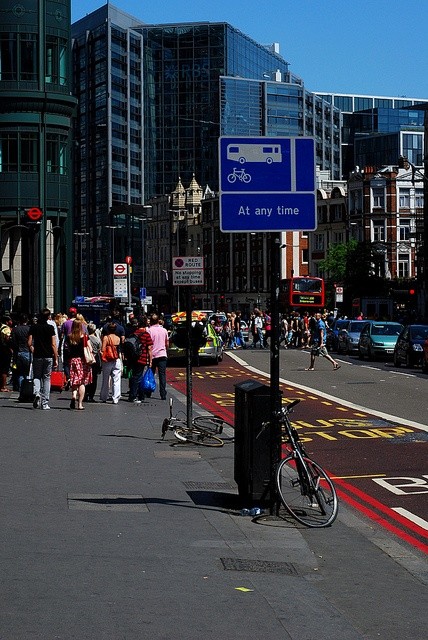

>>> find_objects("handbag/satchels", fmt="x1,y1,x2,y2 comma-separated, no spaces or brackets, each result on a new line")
101,336,118,362
83,334,96,364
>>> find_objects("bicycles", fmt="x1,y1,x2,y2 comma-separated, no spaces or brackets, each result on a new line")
256,399,338,528
161,397,235,447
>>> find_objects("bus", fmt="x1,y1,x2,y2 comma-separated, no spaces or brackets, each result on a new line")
278,276,325,314
72,296,141,328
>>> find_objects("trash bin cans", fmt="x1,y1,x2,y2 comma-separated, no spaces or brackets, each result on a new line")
233,378,284,513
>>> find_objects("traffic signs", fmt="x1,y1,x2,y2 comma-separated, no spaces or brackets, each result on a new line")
220,294,225,307
141,296,152,304
218,135,317,233
114,279,127,297
336,287,343,294
172,257,205,286
140,288,146,299
336,294,343,302
125,256,132,264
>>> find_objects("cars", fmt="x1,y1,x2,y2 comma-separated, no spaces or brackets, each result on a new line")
209,310,227,325
167,321,223,367
334,320,350,338
358,320,404,361
394,325,428,367
240,320,249,341
347,320,375,354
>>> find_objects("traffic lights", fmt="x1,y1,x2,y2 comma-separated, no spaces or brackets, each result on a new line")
408,289,416,295
333,282,338,289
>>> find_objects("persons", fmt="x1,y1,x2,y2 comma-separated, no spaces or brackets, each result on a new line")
305,314,341,370
210,311,312,348
10,314,32,391
0,315,12,392
99,324,123,404
149,315,169,401
101,311,126,398
127,317,154,404
59,306,86,392
83,324,101,401
123,313,138,336
190,317,207,365
355,311,367,320
28,309,58,410
66,319,93,410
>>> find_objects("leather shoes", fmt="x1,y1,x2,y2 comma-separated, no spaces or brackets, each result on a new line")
113,398,118,403
161,396,166,399
78,407,85,410
70,398,75,409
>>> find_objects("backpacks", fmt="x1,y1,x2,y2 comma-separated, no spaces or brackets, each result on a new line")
123,332,146,369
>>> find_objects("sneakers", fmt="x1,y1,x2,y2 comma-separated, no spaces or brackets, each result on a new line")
33,391,41,409
133,399,141,403
42,404,51,409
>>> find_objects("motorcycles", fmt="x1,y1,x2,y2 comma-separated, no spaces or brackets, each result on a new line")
336,328,353,354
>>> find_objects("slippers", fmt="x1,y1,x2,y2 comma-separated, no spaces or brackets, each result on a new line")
333,365,341,371
305,367,315,371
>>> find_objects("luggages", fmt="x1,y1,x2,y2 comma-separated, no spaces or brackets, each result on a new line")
18,379,35,403
50,372,65,394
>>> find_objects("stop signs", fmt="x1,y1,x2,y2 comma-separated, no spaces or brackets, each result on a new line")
114,264,127,276
24,208,43,225
